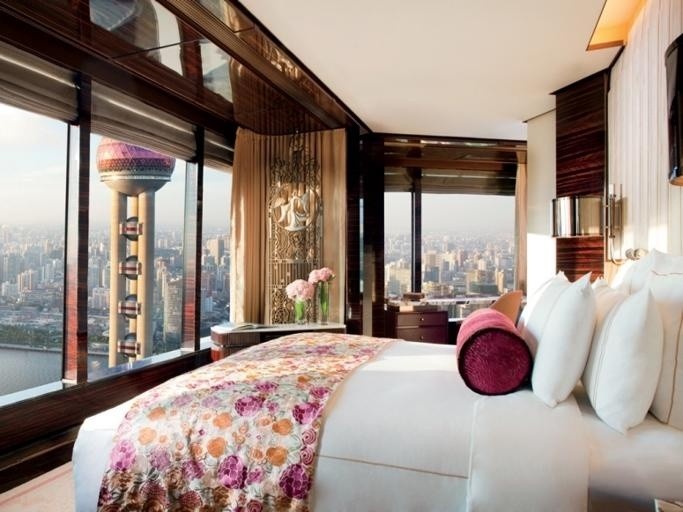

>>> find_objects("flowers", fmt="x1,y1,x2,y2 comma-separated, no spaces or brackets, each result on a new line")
307,268,335,316
285,281,314,320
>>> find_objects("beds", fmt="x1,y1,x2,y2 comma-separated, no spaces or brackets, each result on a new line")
70,246,683,512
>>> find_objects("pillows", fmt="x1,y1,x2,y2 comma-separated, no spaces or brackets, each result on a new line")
517,270,597,407
645,248,683,273
610,260,683,425
457,305,533,397
582,284,663,433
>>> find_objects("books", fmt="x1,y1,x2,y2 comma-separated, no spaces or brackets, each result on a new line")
398,304,440,312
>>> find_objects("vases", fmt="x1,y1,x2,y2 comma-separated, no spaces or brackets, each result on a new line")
293,302,307,324
321,282,331,324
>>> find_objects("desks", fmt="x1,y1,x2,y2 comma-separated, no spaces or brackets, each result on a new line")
209,320,346,359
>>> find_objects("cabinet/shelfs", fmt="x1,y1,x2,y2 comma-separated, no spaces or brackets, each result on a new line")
550,68,609,285
523,106,557,291
386,311,449,344
